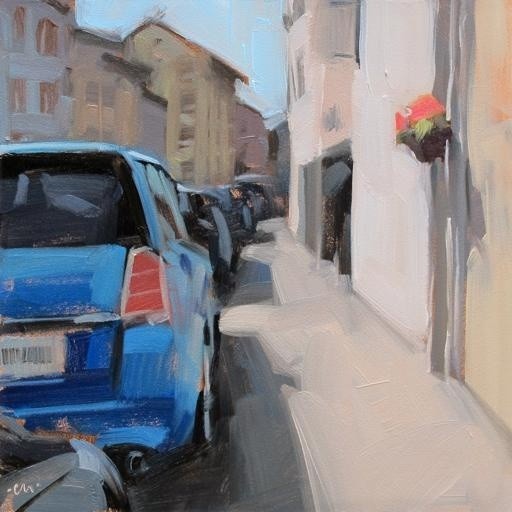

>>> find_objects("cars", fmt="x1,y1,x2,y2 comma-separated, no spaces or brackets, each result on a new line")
0,415,133,512
176,174,287,281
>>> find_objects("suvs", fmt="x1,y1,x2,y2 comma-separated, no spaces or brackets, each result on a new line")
0,139,220,466
